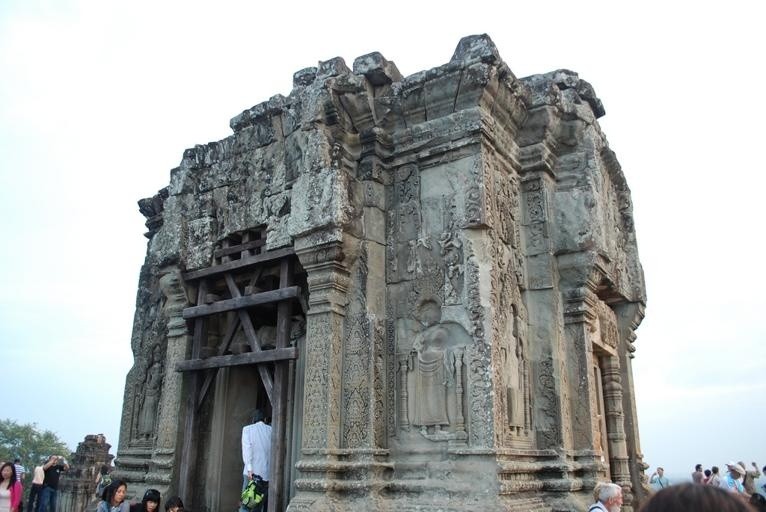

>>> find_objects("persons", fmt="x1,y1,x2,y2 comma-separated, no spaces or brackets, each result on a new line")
587,462,766,512
0,455,186,512
239,408,272,512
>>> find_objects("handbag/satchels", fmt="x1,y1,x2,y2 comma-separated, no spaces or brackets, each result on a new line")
240,472,268,512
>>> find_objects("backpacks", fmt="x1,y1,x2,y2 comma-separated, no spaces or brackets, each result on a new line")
101,472,111,487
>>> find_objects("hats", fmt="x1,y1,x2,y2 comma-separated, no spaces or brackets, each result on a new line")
731,464,746,476
250,409,268,423
